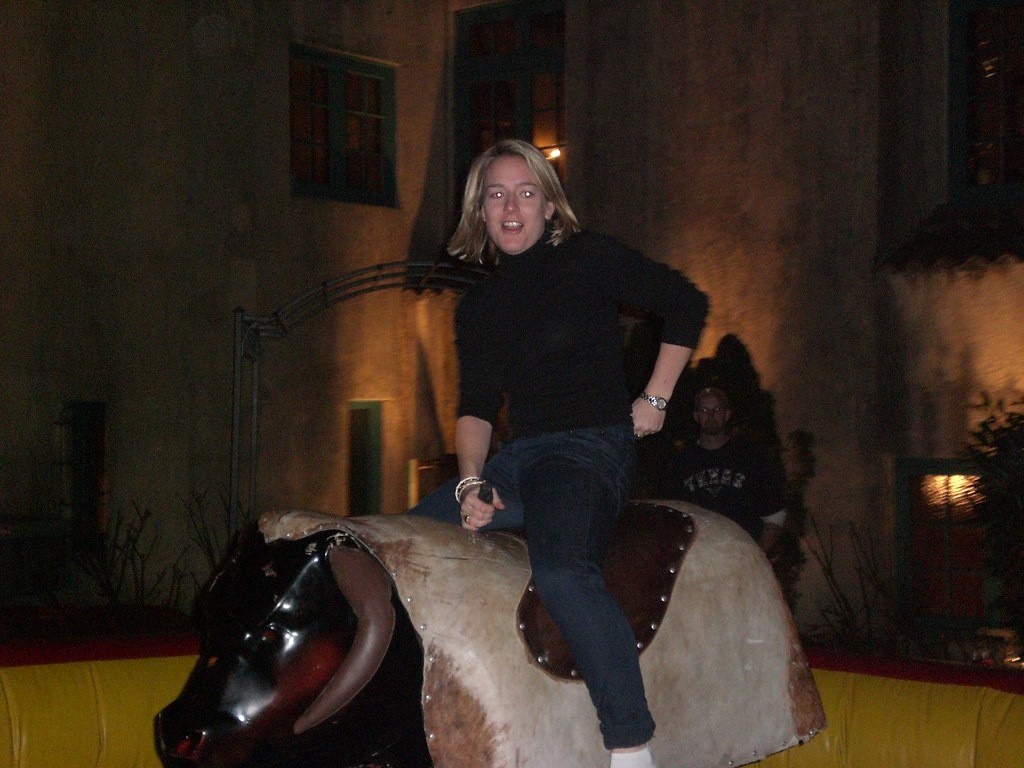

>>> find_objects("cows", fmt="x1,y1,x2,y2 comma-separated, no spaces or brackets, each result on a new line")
154,503,828,768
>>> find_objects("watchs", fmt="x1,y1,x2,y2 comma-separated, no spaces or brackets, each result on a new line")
640,391,668,410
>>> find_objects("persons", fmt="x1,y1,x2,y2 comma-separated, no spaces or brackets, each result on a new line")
655,387,787,586
403,140,709,768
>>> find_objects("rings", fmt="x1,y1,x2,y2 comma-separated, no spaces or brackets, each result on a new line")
463,514,470,523
635,434,640,438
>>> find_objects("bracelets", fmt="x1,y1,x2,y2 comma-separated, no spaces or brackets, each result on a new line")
454,477,486,503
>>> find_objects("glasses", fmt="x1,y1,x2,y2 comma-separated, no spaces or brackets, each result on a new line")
697,407,725,414
